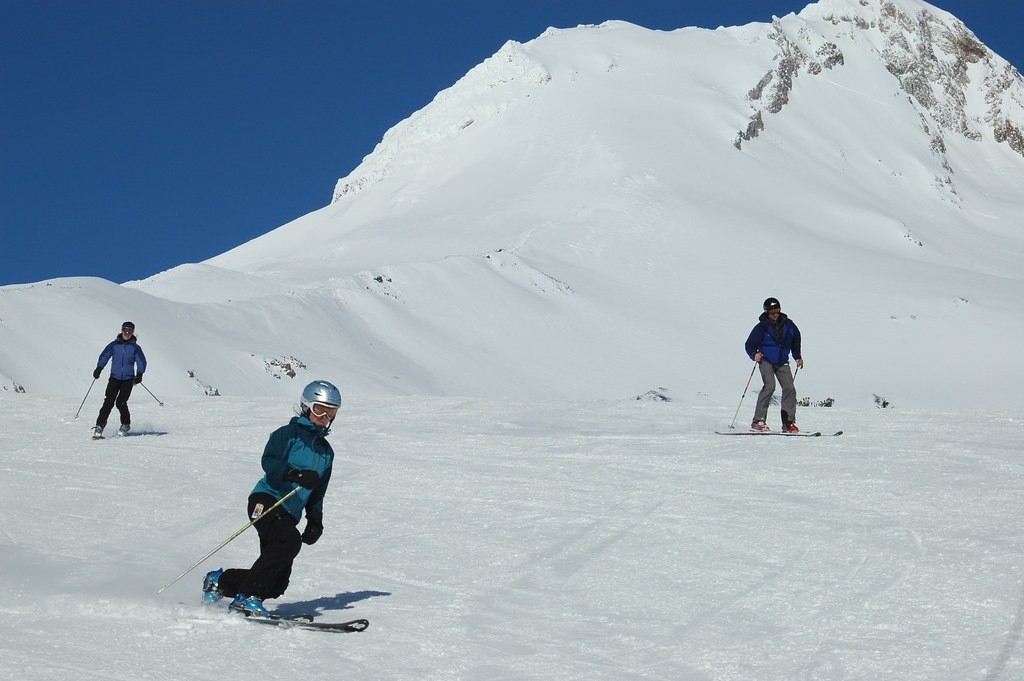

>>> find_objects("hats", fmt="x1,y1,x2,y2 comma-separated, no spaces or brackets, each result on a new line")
122,322,134,334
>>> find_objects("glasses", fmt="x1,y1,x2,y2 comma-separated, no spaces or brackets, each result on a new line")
121,329,133,333
768,308,781,314
307,401,339,421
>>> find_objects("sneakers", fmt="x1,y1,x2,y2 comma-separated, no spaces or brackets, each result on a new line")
201,569,223,606
228,593,278,622
91,426,104,440
118,424,130,436
782,422,800,434
750,419,770,432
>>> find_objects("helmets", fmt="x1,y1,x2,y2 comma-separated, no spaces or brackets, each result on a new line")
762,298,781,317
300,380,342,422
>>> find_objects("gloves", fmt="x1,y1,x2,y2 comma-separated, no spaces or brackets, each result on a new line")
134,373,142,385
287,468,320,491
93,367,102,378
301,520,323,545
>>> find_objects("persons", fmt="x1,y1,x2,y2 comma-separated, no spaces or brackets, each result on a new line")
94,321,147,437
745,297,803,434
203,379,342,616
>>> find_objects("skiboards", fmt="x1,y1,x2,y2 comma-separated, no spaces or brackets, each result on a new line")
714,423,844,438
89,423,131,440
183,566,375,633
